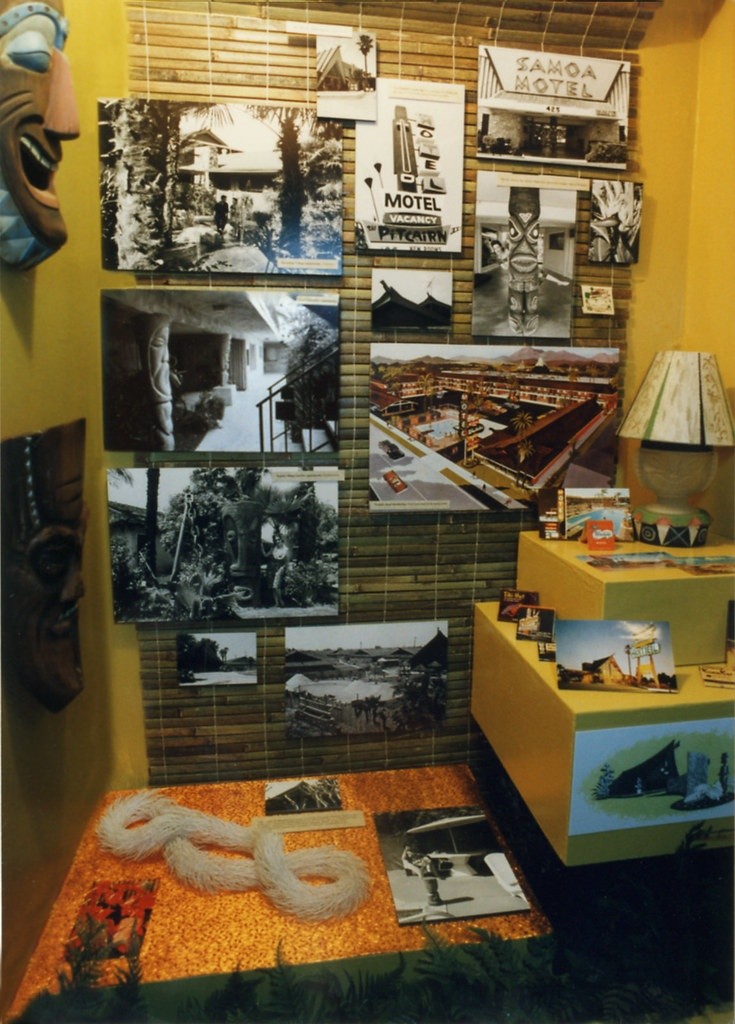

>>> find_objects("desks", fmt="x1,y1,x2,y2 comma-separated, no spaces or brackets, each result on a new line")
468,524,735,867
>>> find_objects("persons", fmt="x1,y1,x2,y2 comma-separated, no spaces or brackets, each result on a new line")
213,195,245,248
1,417,89,716
168,354,182,415
400,842,435,883
258,534,294,608
1,0,81,268
491,239,570,286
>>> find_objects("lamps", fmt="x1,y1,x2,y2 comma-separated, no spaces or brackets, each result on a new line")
615,349,735,548
